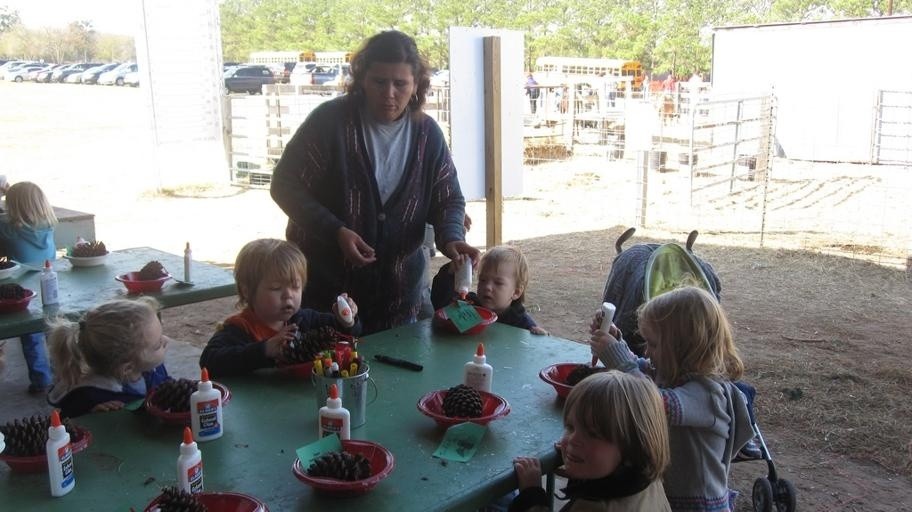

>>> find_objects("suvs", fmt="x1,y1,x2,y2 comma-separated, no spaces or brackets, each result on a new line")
525,74,540,98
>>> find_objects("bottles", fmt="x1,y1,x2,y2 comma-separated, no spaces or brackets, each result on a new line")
454,254,471,300
318,384,351,441
182,241,192,284
190,367,226,441
313,339,360,376
336,294,354,325
463,342,495,392
40,257,61,304
590,303,615,366
176,426,205,494
46,410,78,495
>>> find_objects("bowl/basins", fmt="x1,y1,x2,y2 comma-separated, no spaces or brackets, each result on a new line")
1,424,90,473
117,273,172,293
147,380,231,421
292,441,395,494
415,387,509,428
0,256,21,278
5,287,38,313
277,332,361,379
65,250,111,267
539,360,601,400
145,491,268,511
435,303,497,334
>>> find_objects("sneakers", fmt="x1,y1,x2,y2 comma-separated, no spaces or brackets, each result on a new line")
29,382,54,394
738,439,761,460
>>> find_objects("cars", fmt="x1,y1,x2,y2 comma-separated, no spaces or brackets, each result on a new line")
428,69,449,89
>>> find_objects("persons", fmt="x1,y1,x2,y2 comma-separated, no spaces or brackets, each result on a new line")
200,239,362,377
430,245,550,336
270,31,482,333
508,369,673,512
0,176,59,394
641,70,706,94
526,73,541,115
590,271,756,512
45,294,170,418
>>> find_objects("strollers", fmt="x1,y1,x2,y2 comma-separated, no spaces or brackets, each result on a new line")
598,224,800,512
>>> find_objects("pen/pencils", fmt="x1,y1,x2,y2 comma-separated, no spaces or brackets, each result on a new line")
314,339,363,378
374,355,424,371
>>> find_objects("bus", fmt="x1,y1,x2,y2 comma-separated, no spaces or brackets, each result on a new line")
532,55,643,92
249,50,353,63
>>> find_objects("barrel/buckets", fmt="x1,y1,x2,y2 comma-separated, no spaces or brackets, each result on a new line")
310,362,379,429
